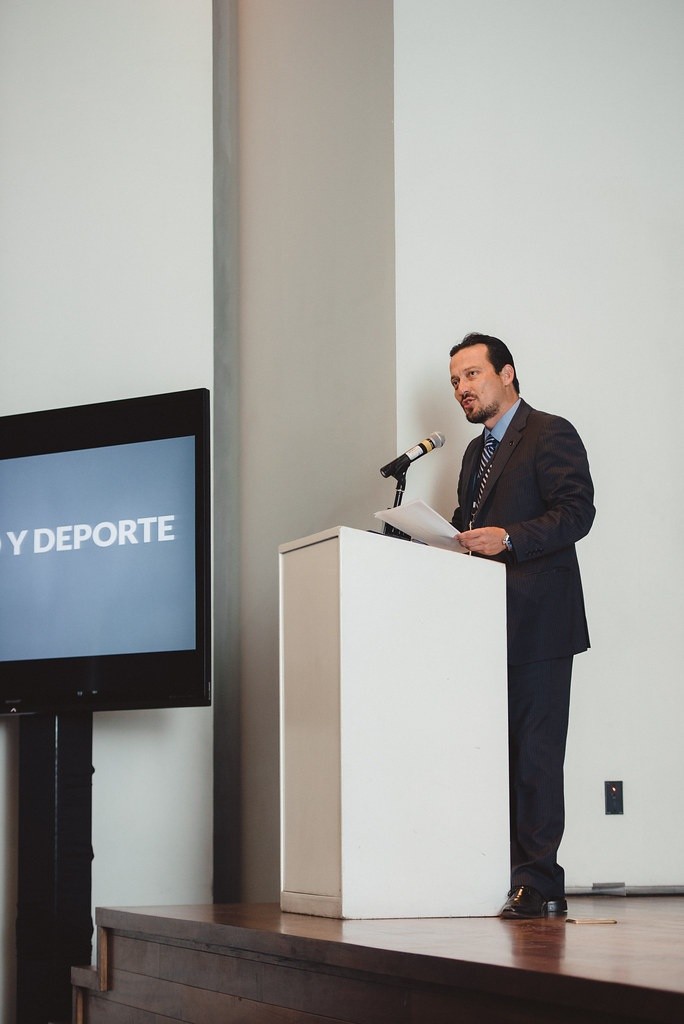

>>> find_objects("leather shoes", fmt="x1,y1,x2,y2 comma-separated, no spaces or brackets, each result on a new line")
548,895,568,912
500,885,548,919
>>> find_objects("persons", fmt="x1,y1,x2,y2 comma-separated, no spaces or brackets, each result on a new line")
449,333,597,920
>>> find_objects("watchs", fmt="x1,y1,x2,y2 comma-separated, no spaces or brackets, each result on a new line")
502,533,515,552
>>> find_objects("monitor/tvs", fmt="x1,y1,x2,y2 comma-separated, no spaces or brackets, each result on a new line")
0,387,212,718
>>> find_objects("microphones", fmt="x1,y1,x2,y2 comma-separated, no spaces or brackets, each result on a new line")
380,432,446,478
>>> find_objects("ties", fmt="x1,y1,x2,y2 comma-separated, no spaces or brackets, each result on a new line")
471,434,497,520
